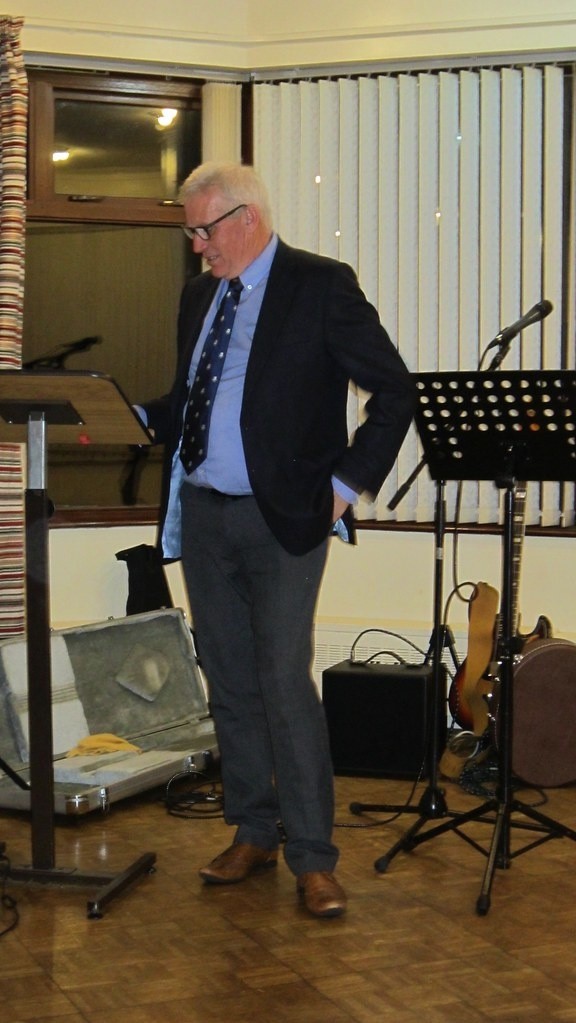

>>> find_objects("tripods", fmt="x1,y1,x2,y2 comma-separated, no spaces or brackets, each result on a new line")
350,326,575,914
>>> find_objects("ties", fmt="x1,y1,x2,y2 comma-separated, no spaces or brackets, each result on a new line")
176,275,246,474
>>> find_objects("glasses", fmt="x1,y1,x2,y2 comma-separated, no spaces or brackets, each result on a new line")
180,203,248,240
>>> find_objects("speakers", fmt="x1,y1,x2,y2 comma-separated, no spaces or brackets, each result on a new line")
322,660,446,781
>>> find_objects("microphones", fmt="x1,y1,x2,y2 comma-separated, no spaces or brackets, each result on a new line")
487,299,554,348
62,334,103,348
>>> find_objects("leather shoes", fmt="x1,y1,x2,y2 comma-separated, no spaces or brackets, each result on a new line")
295,872,348,918
197,843,279,881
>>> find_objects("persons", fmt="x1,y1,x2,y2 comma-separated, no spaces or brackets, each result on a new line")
130,162,419,916
